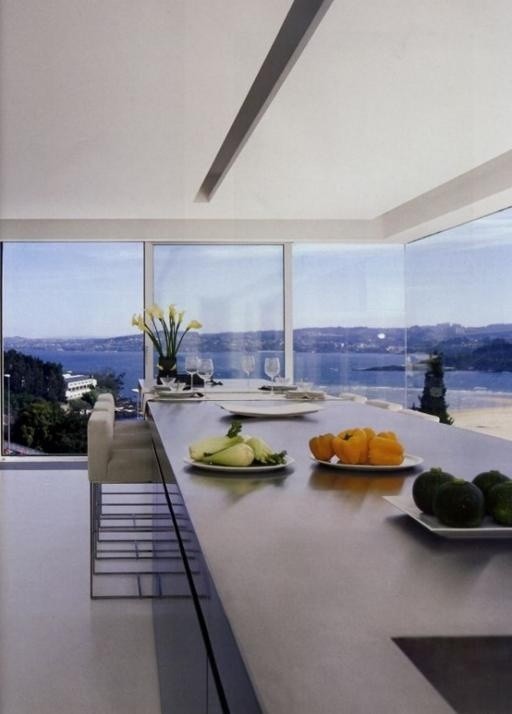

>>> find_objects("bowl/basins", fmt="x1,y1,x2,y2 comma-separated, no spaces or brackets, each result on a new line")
161,377,176,386
168,382,186,391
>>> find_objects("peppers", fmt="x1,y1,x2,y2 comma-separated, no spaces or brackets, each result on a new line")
309,428,405,465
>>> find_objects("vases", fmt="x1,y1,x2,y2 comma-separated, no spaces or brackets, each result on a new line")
157,356,177,385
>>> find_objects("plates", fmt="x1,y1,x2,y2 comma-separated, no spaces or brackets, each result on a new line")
214,400,323,417
158,391,194,397
183,450,295,472
381,494,511,541
309,451,423,471
153,384,170,391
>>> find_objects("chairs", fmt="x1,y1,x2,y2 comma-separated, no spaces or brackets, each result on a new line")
399,409,440,423
364,399,403,412
85,393,187,599
339,392,367,403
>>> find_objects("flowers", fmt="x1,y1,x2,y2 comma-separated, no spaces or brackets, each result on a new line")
131,302,203,357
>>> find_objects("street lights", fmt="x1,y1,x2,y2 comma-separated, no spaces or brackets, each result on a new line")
132,387,140,421
5,373,12,455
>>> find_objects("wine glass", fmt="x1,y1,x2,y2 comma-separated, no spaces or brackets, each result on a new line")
196,358,214,392
263,357,281,398
184,355,199,392
241,355,256,388
